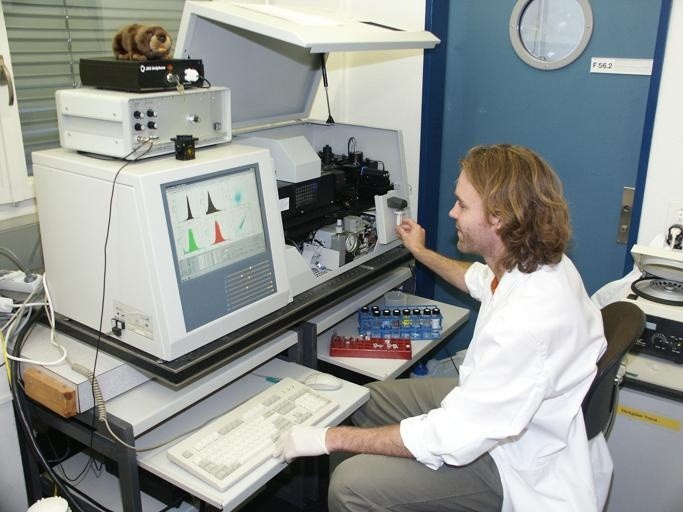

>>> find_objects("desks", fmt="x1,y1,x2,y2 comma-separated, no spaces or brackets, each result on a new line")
19,263,470,512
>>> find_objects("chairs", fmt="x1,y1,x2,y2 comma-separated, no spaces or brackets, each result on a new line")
581,300,646,440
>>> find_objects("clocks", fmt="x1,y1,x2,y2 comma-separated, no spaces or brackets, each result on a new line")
331,232,358,266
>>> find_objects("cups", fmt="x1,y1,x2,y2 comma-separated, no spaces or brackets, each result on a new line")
383,290,407,306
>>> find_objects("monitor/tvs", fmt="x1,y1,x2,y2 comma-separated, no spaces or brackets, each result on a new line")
31,143,294,362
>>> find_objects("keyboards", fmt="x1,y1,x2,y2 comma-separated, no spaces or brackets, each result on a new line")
166,377,339,492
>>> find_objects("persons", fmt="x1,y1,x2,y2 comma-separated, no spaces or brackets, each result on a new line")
269,146,614,512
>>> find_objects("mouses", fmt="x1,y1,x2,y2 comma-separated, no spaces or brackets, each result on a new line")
304,372,343,391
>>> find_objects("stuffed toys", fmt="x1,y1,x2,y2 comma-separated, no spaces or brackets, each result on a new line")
113,22,174,63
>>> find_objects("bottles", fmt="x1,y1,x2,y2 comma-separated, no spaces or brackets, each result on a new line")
331,304,439,353
